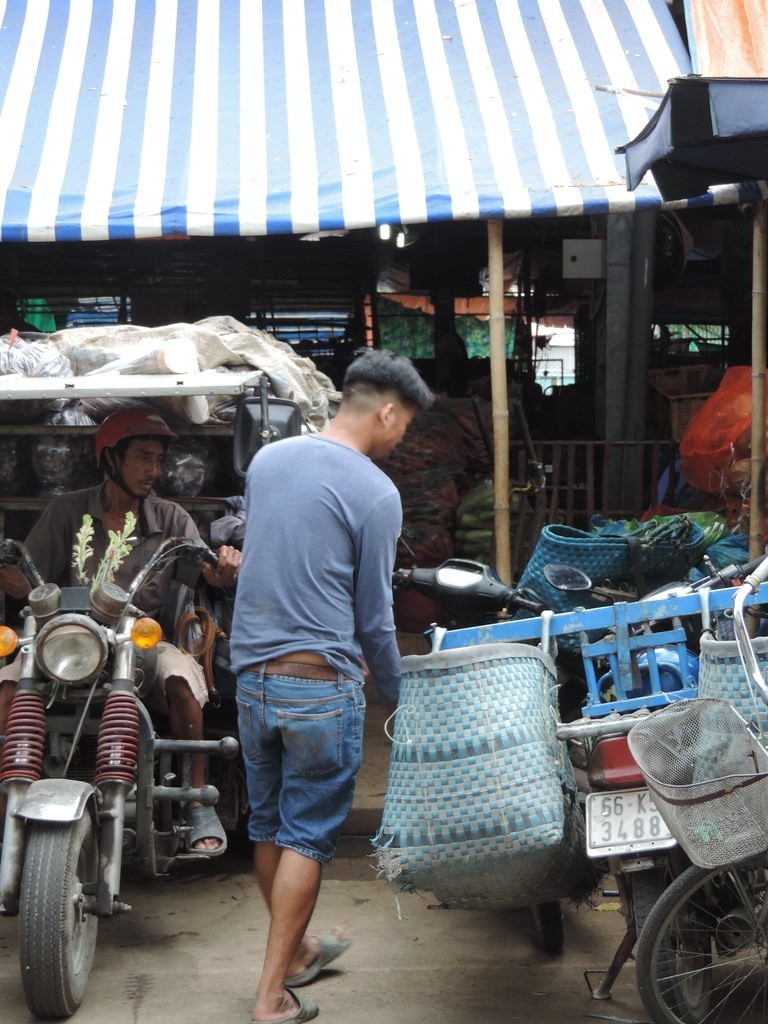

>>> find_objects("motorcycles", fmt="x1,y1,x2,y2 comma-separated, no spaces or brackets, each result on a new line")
1,376,260,1019
540,562,753,1024
391,557,596,957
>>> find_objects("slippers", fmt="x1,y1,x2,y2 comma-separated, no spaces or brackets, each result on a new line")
250,987,319,1024
184,803,228,856
285,933,352,986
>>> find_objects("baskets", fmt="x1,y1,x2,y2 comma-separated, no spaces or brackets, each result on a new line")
627,696,768,870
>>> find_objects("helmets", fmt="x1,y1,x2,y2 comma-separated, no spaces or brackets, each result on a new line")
94,406,178,469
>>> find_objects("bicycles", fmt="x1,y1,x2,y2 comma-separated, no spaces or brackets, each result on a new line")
625,552,768,1024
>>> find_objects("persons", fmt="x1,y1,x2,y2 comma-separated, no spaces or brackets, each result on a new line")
1,408,243,857
229,350,433,1024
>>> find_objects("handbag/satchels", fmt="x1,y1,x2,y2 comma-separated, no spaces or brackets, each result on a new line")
508,516,707,660
693,585,768,785
368,609,606,910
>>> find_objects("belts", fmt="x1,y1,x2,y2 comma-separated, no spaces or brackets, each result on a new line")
244,659,352,681
